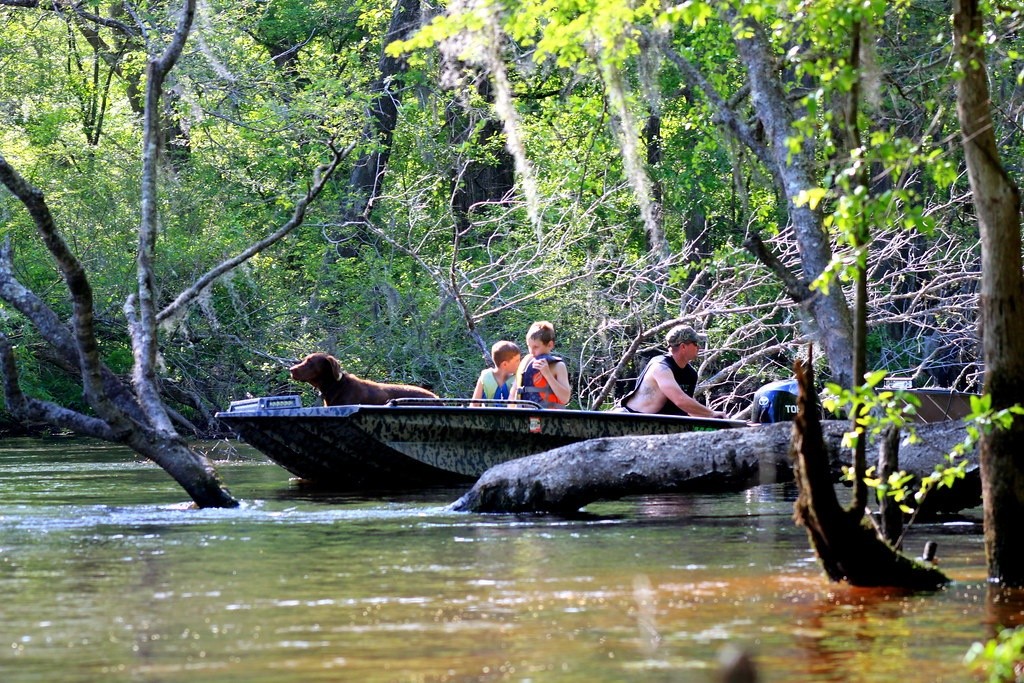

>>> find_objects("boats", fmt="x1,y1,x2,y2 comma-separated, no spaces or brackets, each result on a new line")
873,377,983,425
213,377,822,498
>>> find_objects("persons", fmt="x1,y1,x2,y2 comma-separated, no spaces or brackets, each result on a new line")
610,324,726,418
507,321,570,409
468,341,521,407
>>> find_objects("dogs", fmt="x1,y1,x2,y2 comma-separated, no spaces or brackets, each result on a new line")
289,352,441,406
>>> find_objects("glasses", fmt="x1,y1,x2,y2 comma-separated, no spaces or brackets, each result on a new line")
687,341,698,347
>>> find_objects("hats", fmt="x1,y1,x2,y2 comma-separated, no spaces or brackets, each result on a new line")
666,325,709,347
